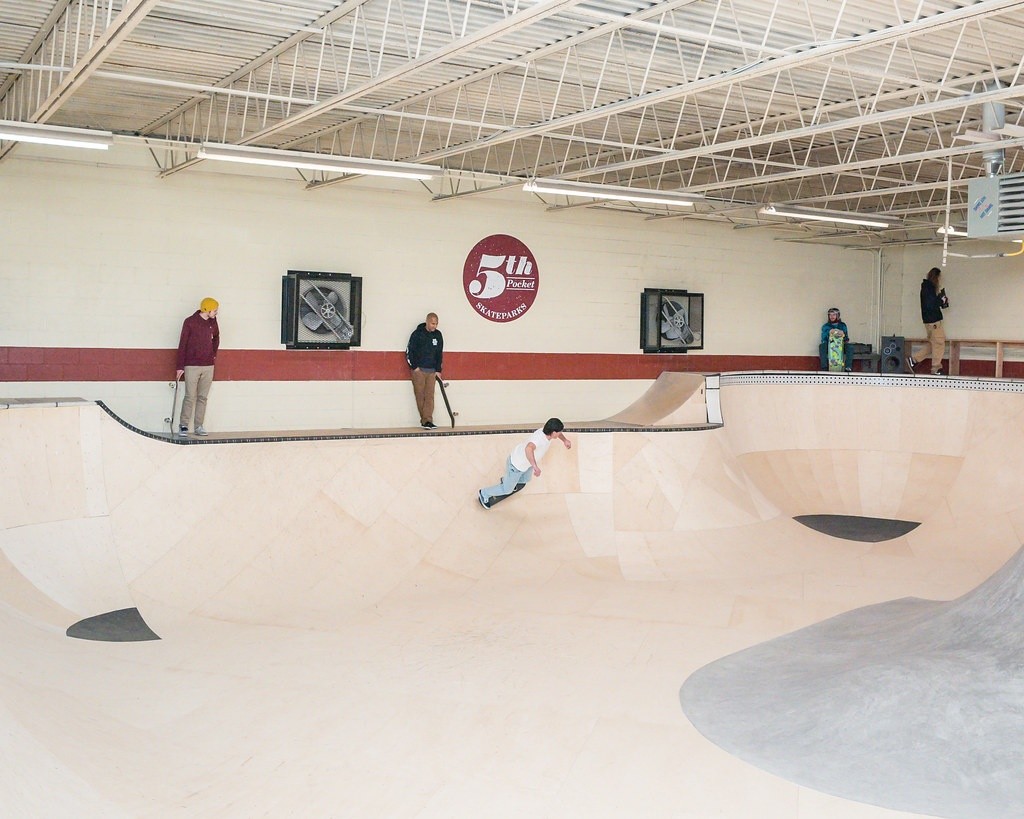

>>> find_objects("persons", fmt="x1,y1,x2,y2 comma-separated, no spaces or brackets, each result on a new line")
175,296,220,438
405,311,444,431
904,267,950,376
818,307,854,372
478,417,572,511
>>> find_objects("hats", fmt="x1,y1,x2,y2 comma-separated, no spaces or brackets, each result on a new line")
828,308,840,314
200,298,219,313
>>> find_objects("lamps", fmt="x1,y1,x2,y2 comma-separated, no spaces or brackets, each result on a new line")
936,225,1024,243
521,178,706,207
196,141,444,181
757,202,902,227
1,118,115,153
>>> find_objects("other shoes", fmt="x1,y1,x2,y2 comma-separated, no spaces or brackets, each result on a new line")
845,367,851,371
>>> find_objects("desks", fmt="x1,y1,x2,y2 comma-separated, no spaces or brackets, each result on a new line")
905,338,1024,378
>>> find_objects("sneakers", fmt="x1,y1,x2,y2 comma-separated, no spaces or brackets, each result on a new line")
931,368,944,375
422,421,437,430
479,489,490,509
906,356,916,374
501,478,518,492
194,427,208,436
178,425,188,436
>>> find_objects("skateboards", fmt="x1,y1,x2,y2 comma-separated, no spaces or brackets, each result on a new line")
827,328,846,372
436,374,459,429
479,483,526,509
163,377,180,437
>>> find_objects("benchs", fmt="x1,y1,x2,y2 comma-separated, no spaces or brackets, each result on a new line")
842,343,881,370
